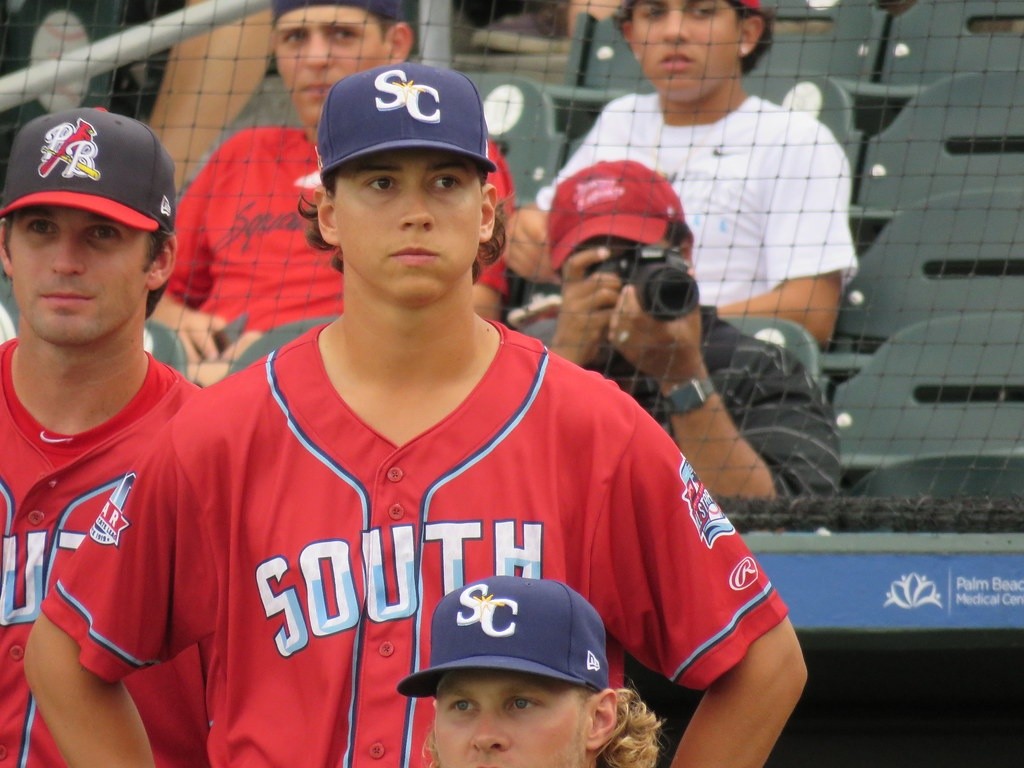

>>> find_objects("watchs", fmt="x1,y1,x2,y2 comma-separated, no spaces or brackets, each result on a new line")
659,374,715,415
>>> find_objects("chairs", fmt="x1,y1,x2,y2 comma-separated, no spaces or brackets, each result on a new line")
143,0,1024,531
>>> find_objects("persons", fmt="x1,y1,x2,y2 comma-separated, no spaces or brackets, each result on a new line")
147,0,517,384
0,103,210,768
503,1,860,349
396,575,663,768
546,158,844,503
22,60,810,768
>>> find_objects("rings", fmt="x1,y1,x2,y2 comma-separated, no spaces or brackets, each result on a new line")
618,331,629,342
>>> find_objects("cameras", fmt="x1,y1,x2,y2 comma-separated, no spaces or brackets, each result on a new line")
589,220,701,321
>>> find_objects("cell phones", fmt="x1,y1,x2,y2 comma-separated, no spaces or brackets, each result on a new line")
213,312,249,354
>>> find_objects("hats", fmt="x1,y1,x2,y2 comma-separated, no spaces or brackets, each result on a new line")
271,0,402,23
315,62,496,187
395,574,610,698
0,105,176,238
549,159,684,271
